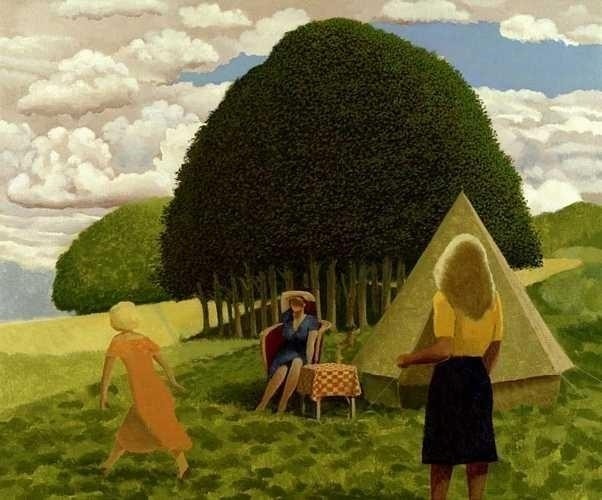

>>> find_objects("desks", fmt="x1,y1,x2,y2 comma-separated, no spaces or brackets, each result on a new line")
300,362,362,421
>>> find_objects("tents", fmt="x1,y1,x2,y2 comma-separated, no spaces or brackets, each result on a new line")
351,189,574,411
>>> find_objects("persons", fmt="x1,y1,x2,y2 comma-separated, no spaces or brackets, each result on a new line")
396,234,504,500
97,300,197,481
254,289,325,414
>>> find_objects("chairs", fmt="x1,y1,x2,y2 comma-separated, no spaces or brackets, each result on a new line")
262,318,332,414
